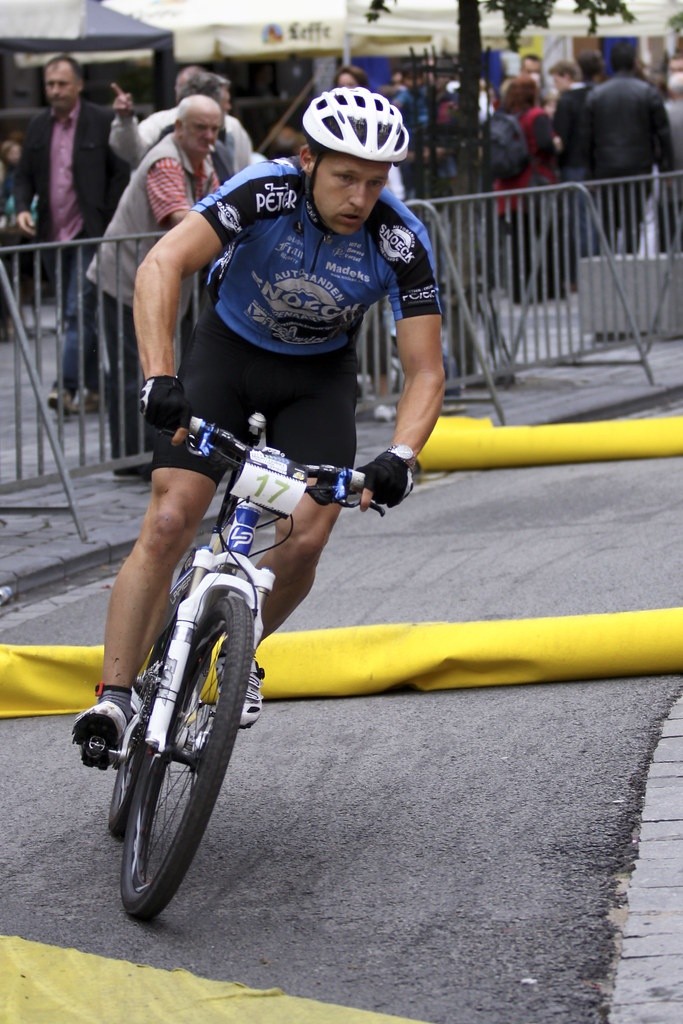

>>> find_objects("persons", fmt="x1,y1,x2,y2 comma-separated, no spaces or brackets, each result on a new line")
0,36,683,484
79,86,446,771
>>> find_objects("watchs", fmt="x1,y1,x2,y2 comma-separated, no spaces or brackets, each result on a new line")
387,444,417,474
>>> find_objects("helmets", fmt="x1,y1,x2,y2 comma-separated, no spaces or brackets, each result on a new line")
302,86,410,162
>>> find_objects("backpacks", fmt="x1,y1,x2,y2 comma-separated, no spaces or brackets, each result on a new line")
481,110,527,181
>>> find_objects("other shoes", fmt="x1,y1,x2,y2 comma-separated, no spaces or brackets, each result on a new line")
72,396,98,413
48,387,72,415
114,463,152,475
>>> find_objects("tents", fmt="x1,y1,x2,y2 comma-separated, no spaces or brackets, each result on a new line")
0,0,683,113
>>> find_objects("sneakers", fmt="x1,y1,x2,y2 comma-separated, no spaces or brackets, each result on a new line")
71,701,129,770
216,648,265,729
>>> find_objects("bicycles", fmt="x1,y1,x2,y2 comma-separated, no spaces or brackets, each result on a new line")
81,412,386,923
